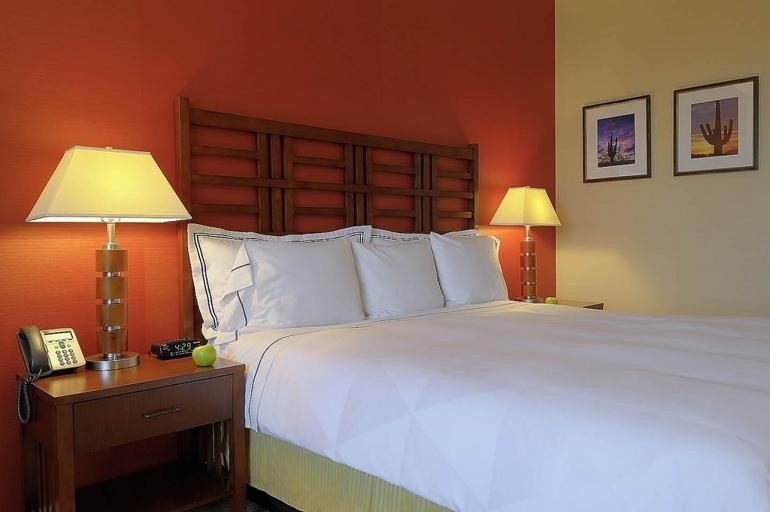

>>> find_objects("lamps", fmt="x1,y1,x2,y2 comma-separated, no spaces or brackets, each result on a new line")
24,143,192,370
489,185,562,303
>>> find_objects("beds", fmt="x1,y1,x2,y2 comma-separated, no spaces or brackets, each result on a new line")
175,92,770,512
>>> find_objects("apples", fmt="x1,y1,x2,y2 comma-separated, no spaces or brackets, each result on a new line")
191,344,217,367
544,296,559,305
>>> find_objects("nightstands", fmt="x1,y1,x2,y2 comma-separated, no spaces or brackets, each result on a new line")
544,299,604,311
17,349,249,512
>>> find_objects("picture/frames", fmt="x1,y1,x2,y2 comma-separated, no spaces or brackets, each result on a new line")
582,95,651,183
673,75,760,176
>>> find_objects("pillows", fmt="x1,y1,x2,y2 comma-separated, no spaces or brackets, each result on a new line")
429,230,510,308
353,238,446,321
239,242,367,331
373,228,481,240
186,222,372,342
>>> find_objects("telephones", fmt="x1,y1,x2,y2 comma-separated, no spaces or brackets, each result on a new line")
16,324,86,378
